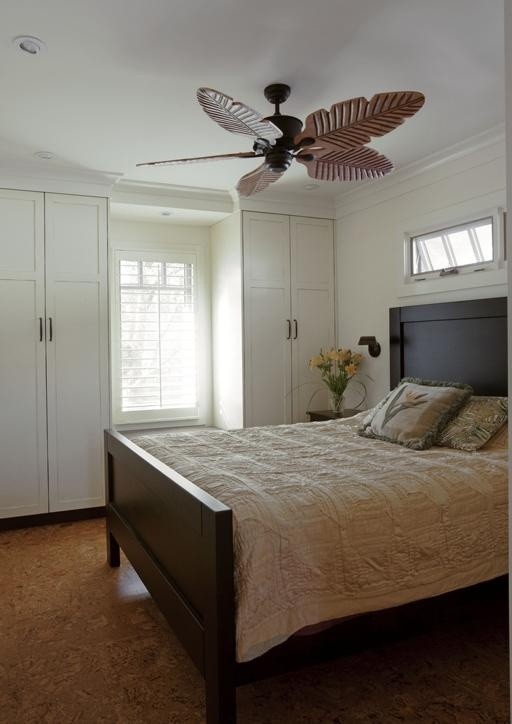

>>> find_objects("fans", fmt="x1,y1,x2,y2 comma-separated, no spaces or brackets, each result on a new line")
132,74,436,201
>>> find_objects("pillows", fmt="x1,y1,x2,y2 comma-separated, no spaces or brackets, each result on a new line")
359,372,474,450
436,391,508,451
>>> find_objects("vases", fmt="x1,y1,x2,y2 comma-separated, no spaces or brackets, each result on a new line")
329,391,346,416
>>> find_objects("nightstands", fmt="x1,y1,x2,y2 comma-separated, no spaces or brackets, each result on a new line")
306,409,361,421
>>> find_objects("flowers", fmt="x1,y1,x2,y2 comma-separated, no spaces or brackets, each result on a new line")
306,345,368,410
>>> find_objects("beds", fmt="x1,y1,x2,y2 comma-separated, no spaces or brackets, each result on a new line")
101,295,510,722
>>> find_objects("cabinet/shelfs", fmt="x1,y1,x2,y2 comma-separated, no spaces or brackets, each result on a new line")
0,179,125,527
204,207,341,429
